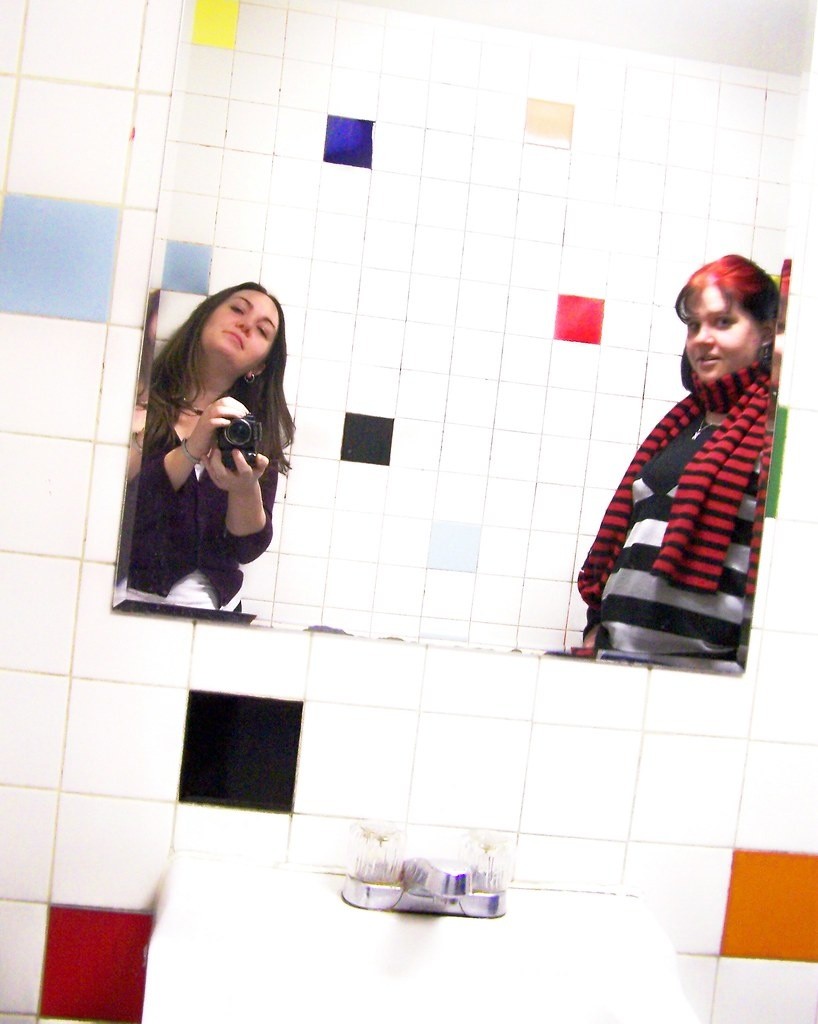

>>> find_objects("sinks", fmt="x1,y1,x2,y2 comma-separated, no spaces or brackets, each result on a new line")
140,909,691,1024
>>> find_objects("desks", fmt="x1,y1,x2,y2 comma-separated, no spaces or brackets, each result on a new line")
134,854,694,1024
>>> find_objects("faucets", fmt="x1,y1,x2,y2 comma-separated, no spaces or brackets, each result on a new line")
417,854,473,904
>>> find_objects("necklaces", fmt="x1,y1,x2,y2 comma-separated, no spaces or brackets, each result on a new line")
690,413,728,438
183,396,203,415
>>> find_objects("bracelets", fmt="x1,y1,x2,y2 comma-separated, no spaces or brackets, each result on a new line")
182,439,202,467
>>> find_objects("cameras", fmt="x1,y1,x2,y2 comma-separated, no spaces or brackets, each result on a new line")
217,414,262,472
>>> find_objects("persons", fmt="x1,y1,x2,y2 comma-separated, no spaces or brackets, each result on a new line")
577,255,778,674
120,282,297,610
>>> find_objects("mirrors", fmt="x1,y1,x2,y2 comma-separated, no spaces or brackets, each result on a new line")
114,0,818,681
